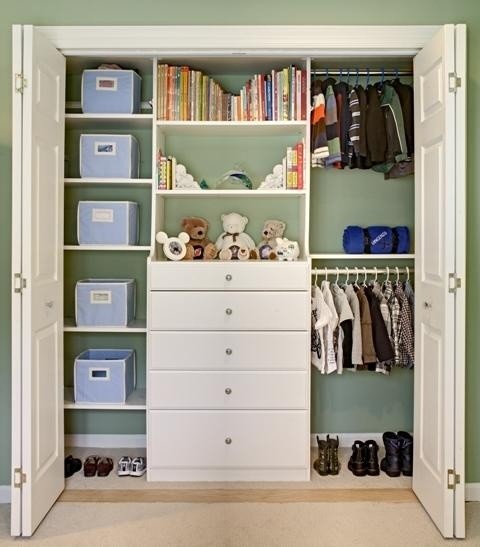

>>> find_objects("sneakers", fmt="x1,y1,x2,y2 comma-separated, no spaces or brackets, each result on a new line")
96,456,114,477
116,455,133,476
130,456,146,477
83,453,100,477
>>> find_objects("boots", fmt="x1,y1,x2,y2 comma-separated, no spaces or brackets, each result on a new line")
327,434,340,475
313,435,330,476
348,439,366,477
397,431,413,477
364,439,380,476
380,431,402,477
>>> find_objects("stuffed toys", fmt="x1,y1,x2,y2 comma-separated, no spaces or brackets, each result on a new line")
273,237,300,261
181,216,215,260
212,212,256,260
256,218,287,260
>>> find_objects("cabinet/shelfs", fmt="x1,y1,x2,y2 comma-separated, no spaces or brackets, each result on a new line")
9,22,468,542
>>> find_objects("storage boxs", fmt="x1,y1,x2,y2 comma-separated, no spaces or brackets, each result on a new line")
79,132,138,183
76,200,139,245
73,349,136,405
80,67,143,116
74,279,135,329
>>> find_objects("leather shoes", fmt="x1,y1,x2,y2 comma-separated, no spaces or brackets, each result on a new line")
64,454,81,478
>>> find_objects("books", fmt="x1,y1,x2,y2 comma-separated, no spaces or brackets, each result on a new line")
156,147,171,190
282,138,306,190
157,63,307,121
